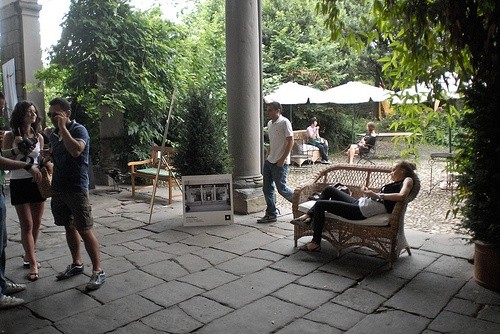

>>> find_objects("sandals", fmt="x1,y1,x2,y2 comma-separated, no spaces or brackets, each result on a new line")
296,242,322,252
290,214,312,227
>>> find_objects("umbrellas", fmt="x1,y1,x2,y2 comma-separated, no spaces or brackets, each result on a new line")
392,67,487,105
310,81,395,144
262,81,321,125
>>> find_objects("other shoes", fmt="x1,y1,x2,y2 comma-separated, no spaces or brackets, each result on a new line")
85,269,106,290
321,160,329,164
55,262,85,280
326,160,332,164
257,212,277,223
0,293,24,306
1,283,26,295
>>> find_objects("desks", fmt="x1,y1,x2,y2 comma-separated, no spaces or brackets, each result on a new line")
357,132,421,159
429,153,458,197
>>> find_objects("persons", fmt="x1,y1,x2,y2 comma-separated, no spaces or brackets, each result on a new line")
257,101,294,223
306,117,332,164
346,122,377,165
290,160,416,252
3,100,53,281
0,91,42,306
42,97,105,291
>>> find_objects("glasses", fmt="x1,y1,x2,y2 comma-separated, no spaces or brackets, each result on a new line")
47,111,61,118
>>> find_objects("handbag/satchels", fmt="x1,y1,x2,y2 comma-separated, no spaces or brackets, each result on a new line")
35,166,53,199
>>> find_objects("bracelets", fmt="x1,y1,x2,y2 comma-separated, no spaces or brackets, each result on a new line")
380,193,384,201
20,156,34,171
11,149,18,155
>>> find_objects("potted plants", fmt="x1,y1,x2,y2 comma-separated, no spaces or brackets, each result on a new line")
366,0,500,289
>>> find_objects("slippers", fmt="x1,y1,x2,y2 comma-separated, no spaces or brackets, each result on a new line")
29,268,39,281
23,258,41,269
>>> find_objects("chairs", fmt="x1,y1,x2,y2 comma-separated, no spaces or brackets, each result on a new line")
357,142,376,165
292,137,319,175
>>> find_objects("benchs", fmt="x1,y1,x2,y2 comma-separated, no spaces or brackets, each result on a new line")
128,147,182,205
289,129,319,164
293,163,421,264
